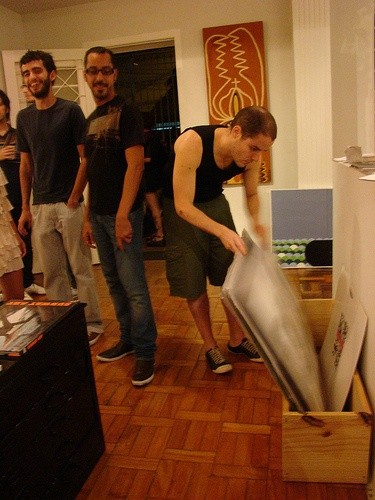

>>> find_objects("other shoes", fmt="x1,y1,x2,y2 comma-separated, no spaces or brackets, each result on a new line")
25,283,46,294
146,235,165,246
23,291,33,300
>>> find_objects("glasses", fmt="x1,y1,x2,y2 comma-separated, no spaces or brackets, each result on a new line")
86,66,115,76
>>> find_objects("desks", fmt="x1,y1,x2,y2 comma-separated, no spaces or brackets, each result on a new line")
0,300,105,500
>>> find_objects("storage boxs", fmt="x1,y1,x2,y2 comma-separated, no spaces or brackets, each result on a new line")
288,269,331,347
281,370,372,483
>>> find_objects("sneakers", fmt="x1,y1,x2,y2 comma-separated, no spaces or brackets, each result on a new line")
132,359,155,386
225,337,264,362
96,341,135,362
88,332,101,345
206,346,232,374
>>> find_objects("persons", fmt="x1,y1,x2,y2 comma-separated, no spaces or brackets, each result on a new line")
82,47,157,386
159,106,276,374
15,50,104,344
0,89,34,288
140,111,165,246
0,167,26,300
